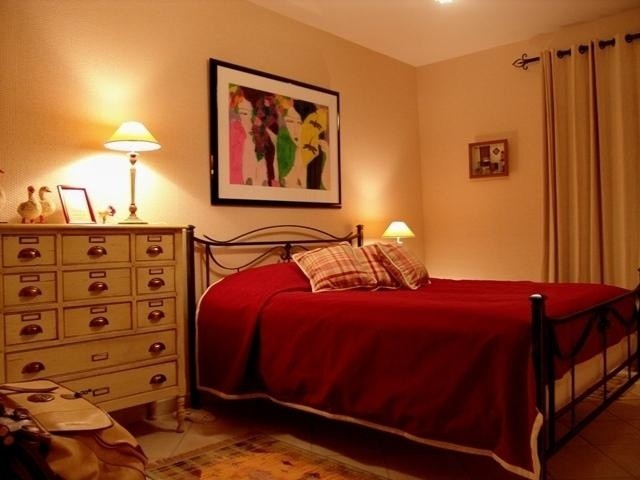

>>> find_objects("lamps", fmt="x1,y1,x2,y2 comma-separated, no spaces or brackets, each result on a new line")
375,218,416,246
98,119,165,226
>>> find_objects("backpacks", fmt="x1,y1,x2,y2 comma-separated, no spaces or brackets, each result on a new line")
0,377,149,480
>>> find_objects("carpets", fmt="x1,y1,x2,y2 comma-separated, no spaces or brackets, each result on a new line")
134,425,402,480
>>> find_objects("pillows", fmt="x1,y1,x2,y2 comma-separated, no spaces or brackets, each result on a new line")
287,239,379,292
348,241,404,293
371,236,433,291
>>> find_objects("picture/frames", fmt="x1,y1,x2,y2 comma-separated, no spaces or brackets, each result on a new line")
467,137,511,180
197,54,344,212
56,184,97,225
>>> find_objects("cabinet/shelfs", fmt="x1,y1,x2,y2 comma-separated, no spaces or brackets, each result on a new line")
0,214,193,438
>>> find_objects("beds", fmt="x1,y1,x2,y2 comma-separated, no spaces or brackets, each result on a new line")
186,218,640,480
